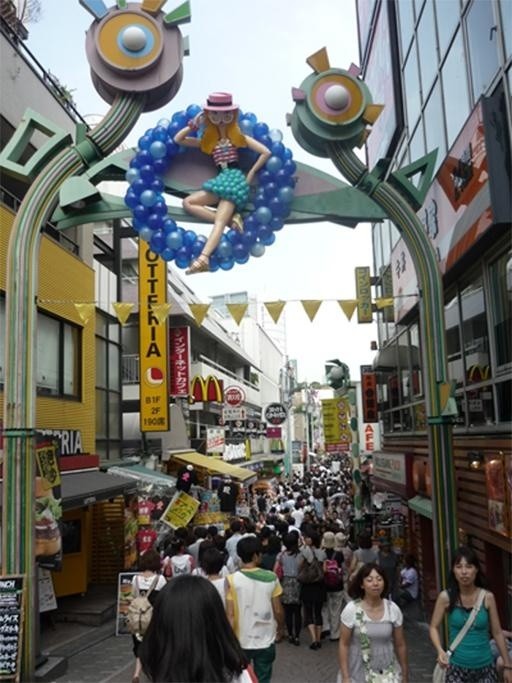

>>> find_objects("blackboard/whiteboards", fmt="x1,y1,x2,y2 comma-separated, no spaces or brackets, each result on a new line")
116,572,144,636
0,573,28,680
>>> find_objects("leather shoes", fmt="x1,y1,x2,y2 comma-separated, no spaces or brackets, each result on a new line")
288,635,299,645
309,641,321,650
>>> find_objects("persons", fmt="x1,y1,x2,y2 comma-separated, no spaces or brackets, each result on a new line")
496,629,512,680
130,447,420,683
428,546,512,683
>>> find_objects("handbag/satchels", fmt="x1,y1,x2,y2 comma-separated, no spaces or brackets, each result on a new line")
275,562,283,580
432,651,451,683
364,662,403,683
297,559,321,583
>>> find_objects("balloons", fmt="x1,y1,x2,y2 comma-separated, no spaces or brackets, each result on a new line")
124,104,296,272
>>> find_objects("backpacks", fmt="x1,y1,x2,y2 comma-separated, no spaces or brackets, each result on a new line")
322,560,344,590
126,589,153,635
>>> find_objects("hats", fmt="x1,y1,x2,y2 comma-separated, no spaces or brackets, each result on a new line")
322,532,336,548
335,533,347,547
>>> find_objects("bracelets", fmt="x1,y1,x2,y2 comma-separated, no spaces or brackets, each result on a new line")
503,666,512,671
